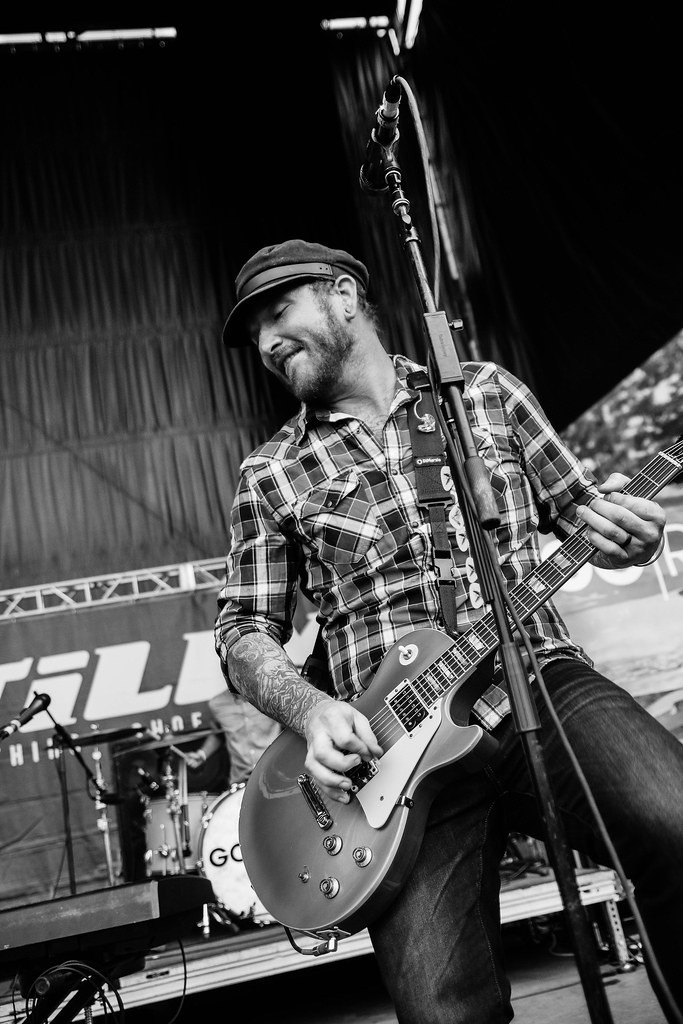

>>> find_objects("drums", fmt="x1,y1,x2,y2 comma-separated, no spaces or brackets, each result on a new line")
199,781,279,926
141,792,218,881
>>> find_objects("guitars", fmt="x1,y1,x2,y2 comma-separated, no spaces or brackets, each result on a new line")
237,438,683,935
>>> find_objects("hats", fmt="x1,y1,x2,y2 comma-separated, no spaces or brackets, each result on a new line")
222,239,368,352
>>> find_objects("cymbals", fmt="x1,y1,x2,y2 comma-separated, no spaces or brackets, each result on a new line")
110,725,226,761
56,725,149,748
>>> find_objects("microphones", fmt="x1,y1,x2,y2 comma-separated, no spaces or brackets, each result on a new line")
1,694,51,739
359,78,403,197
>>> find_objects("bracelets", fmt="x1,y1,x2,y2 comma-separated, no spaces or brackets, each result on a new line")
633,532,665,567
197,750,206,761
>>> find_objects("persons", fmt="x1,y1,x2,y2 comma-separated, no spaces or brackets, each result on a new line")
184,687,282,783
213,240,683,1024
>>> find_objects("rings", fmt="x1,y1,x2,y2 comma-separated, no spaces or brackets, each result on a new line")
620,533,632,549
